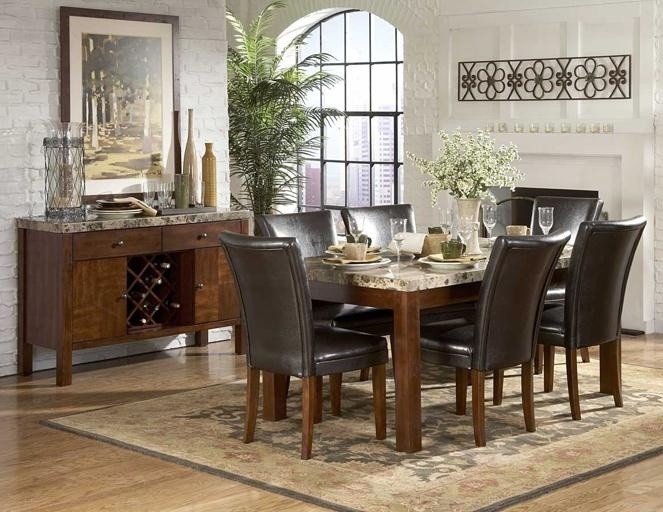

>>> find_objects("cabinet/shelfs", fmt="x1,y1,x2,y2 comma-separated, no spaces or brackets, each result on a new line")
14,203,255,387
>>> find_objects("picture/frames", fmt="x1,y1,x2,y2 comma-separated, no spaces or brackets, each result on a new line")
60,6,183,206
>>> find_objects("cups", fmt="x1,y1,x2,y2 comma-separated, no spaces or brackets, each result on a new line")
440,241,467,259
143,182,155,209
506,225,531,236
174,173,189,208
428,227,450,235
347,235,372,248
342,242,367,261
484,122,614,134
194,181,206,208
157,182,173,209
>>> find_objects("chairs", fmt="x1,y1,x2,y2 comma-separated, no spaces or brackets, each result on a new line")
525,197,603,247
254,210,394,416
420,228,571,447
538,216,646,424
219,229,388,459
341,202,476,325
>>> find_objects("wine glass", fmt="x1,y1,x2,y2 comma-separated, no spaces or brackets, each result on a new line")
347,215,365,242
437,204,497,250
538,207,555,235
389,218,408,273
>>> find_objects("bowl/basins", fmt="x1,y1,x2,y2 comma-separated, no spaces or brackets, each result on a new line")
419,233,448,258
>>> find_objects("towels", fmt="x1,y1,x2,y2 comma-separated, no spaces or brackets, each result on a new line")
388,227,431,254
322,253,382,269
328,241,345,253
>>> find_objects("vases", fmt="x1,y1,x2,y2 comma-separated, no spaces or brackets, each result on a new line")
455,199,484,255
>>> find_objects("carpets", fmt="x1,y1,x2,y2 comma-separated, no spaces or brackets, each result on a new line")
39,354,663,510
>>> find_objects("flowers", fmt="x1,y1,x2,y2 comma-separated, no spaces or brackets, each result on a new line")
406,128,526,206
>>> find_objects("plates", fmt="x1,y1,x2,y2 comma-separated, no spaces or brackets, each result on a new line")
321,246,391,270
417,256,473,270
86,199,143,219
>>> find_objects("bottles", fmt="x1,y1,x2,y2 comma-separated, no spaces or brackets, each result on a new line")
130,260,181,325
183,108,199,206
202,143,218,207
